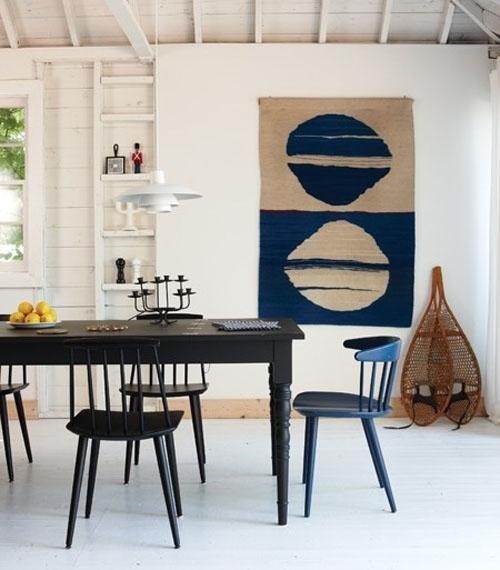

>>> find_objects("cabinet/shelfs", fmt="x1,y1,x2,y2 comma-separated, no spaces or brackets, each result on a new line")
90,52,169,320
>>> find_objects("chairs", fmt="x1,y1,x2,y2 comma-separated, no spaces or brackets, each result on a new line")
60,334,187,553
0,310,34,486
118,309,212,486
291,333,405,519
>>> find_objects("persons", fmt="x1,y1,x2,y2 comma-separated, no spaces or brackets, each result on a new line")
132,143,143,172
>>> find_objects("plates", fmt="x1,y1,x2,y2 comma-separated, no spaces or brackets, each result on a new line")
6,321,61,329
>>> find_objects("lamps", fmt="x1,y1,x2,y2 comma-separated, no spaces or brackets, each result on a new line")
112,1,207,220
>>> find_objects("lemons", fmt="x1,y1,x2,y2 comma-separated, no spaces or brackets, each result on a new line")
10,302,58,323
